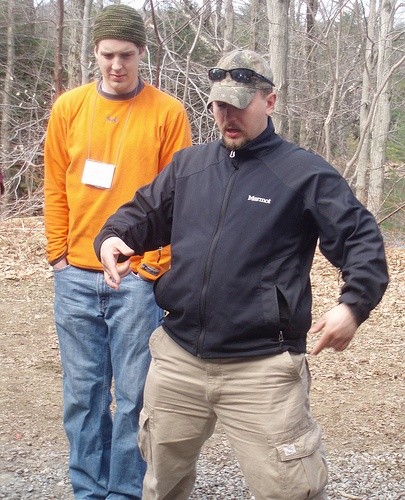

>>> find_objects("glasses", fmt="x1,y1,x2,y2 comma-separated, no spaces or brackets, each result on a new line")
208,67,275,87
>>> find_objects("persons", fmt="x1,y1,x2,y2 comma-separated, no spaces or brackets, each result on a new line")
92,49,389,500
45,4,193,500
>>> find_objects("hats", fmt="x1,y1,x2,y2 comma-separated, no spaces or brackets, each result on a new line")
93,4,147,46
206,49,274,110
